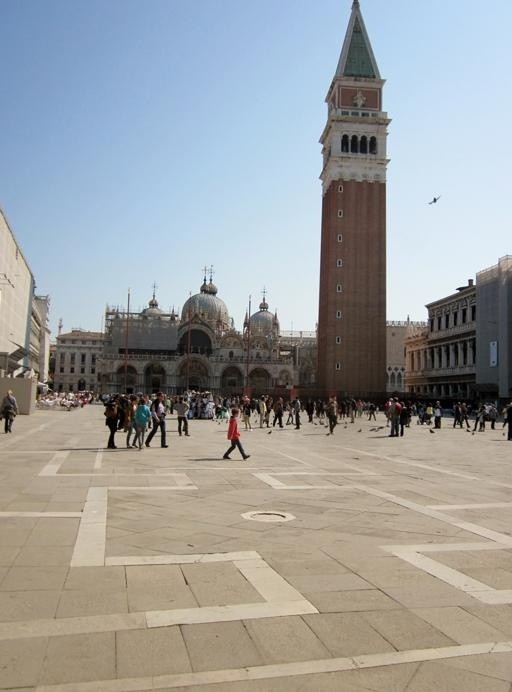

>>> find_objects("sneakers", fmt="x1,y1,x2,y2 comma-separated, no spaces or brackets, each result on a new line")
106,434,191,448
243,454,251,460
223,455,231,459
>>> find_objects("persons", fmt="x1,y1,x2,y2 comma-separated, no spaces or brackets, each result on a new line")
223,409,251,460
0,390,18,433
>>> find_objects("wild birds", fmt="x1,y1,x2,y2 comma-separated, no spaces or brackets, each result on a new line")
428,196,440,204
